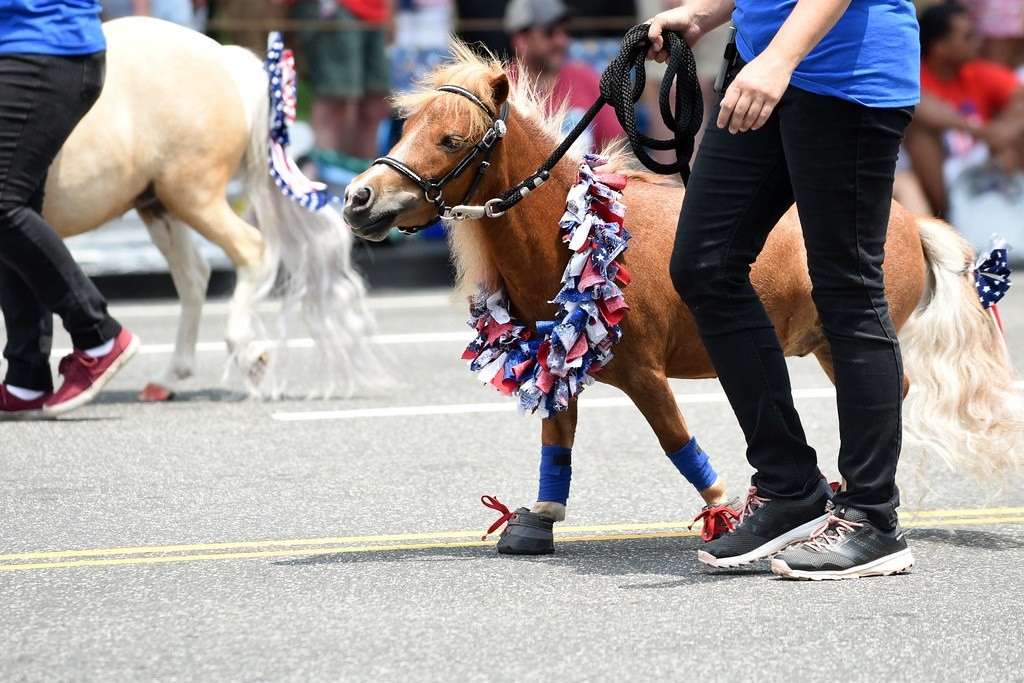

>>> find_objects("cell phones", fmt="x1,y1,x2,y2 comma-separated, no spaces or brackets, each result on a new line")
713,26,736,93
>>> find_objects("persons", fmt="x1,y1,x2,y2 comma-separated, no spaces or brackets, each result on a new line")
0,0,143,423
633,0,922,581
133,0,213,41
325,0,1024,272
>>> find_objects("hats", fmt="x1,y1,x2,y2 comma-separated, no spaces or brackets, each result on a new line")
503,0,568,33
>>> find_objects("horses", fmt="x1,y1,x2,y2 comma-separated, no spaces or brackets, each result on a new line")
0,15,401,408
341,36,1024,557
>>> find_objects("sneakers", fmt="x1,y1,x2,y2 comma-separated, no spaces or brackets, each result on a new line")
0,380,55,415
42,325,141,417
696,472,839,568
771,502,913,581
